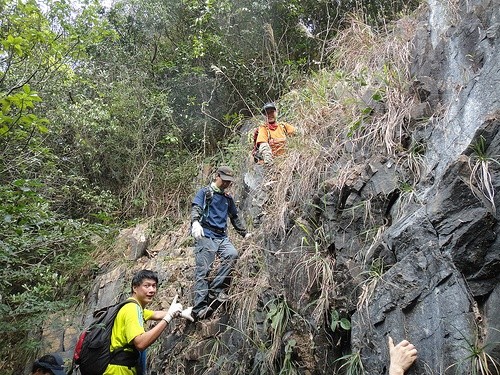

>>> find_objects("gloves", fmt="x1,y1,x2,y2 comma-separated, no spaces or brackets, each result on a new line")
245,232,255,241
179,306,195,322
191,220,204,241
163,294,183,323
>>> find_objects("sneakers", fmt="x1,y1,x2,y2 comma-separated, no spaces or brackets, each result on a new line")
208,291,231,302
196,307,214,319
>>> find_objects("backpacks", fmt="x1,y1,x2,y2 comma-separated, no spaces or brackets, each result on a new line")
252,123,286,164
75,298,141,375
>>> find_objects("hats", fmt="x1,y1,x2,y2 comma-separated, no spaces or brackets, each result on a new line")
216,166,236,184
33,354,67,375
261,103,275,112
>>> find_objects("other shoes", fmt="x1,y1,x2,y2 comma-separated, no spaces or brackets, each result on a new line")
252,215,261,227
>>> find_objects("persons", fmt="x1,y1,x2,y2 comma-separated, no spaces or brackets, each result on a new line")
190,166,252,321
252,101,298,227
388,336,418,375
101,269,196,375
30,352,65,375
